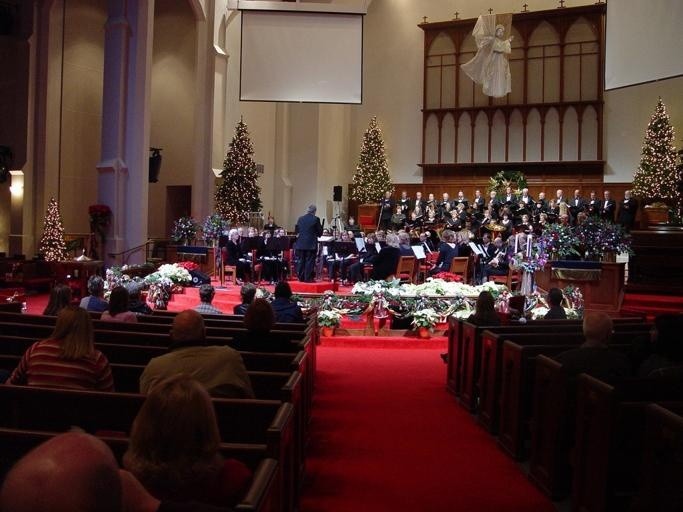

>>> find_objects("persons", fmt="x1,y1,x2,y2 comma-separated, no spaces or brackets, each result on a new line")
2,305,119,393
138,308,259,400
270,281,304,323
540,287,569,321
264,217,278,232
99,286,138,324
233,283,257,314
222,227,286,283
192,283,224,314
438,290,501,366
124,280,153,314
556,311,630,358
120,370,256,479
231,299,290,355
348,189,548,285
315,229,353,281
43,284,75,315
77,274,108,312
294,205,323,282
549,188,639,230
504,293,528,315
0,425,189,508
459,14,515,98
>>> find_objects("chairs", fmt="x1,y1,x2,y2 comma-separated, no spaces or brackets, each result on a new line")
211,241,297,288
337,253,522,293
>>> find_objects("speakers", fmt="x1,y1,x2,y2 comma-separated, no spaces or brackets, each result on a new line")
190,270,210,284
148,155,161,182
333,186,342,201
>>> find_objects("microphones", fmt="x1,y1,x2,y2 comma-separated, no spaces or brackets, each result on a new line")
359,245,365,253
334,215,341,219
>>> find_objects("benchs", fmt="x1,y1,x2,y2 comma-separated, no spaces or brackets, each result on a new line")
443,315,681,512
1,307,319,510
